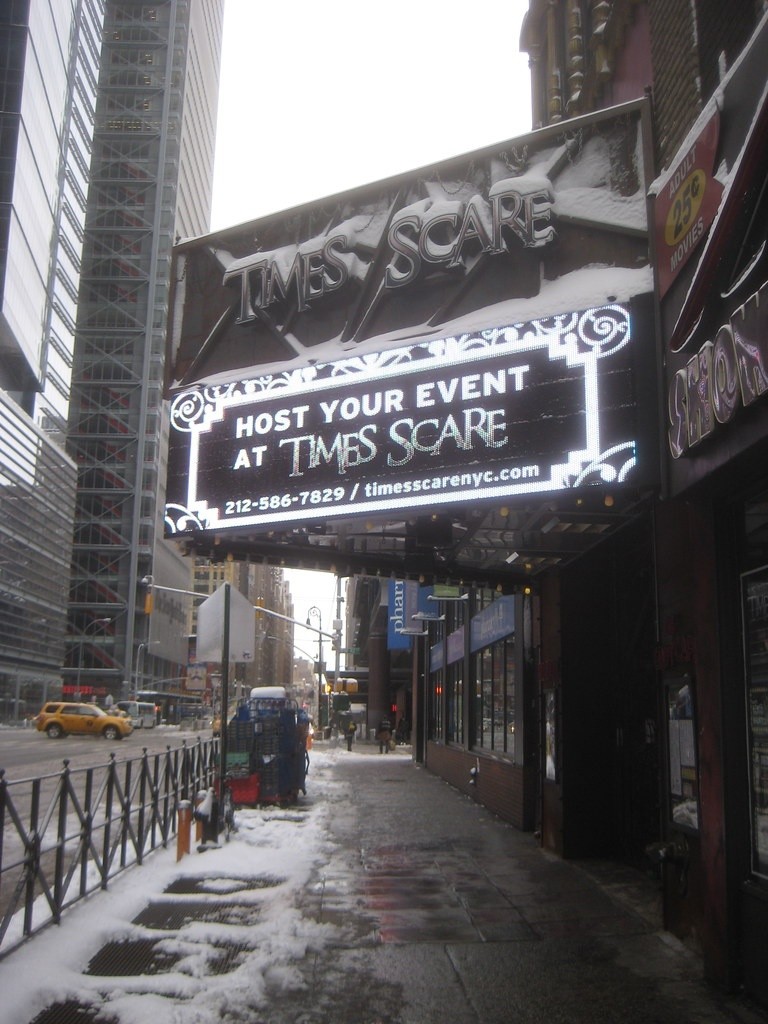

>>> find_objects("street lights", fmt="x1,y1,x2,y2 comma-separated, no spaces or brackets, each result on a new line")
133,643,145,701
76,618,111,703
305,606,323,731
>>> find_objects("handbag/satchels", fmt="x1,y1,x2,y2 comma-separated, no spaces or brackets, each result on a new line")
389,739,396,751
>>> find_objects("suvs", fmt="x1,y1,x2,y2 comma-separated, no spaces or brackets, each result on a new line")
34,701,134,740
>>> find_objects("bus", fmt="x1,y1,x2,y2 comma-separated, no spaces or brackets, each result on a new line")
135,690,204,726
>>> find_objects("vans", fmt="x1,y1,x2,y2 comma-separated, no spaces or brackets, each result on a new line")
106,700,157,729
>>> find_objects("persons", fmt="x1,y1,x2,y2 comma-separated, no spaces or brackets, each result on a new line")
397,716,409,745
342,712,356,752
373,713,392,754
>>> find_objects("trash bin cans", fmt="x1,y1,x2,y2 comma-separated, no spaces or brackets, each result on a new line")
323,726,332,739
